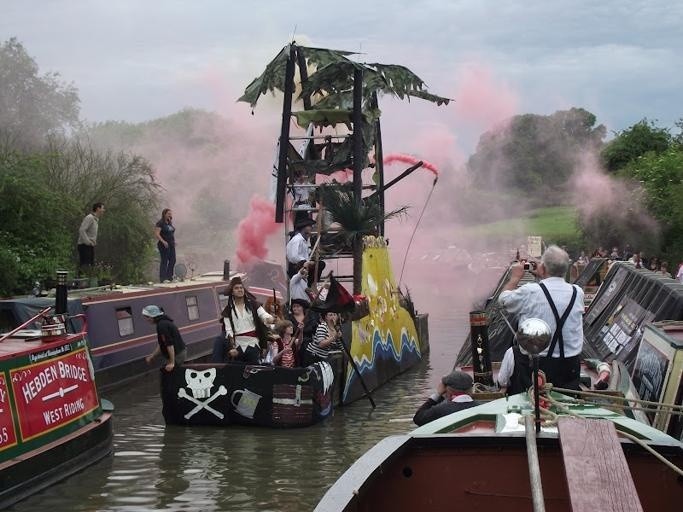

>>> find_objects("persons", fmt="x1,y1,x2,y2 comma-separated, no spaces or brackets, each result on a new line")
142,304,187,372
499,345,532,397
77,202,106,279
413,370,481,427
156,209,176,283
498,244,585,398
221,171,350,368
577,245,683,285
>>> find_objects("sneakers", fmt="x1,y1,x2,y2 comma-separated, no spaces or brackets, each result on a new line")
162,279,171,283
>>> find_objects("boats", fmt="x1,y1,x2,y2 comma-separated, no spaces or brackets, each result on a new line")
159,40,431,425
0,259,286,394
2,306,117,511
310,319,683,512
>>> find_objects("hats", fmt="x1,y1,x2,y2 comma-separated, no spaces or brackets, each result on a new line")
141,304,164,318
442,371,473,390
296,217,316,228
223,276,242,296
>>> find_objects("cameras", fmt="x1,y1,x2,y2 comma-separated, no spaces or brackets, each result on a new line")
523,263,532,272
